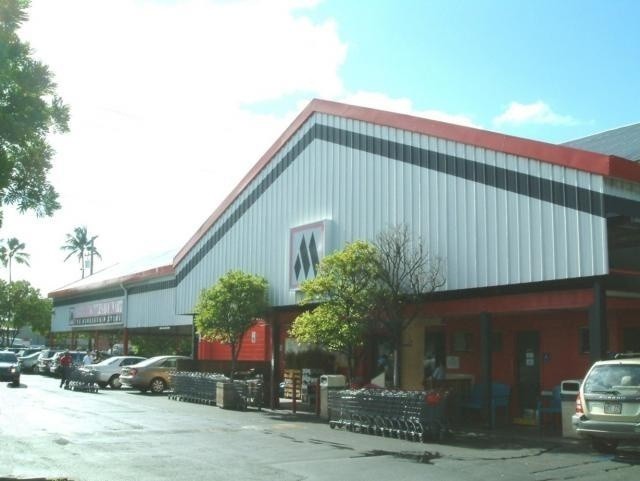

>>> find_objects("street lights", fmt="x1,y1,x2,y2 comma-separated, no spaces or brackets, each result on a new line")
86,234,97,350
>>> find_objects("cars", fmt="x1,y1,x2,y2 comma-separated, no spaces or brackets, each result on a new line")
80,355,146,390
118,354,192,395
0,336,45,375
0,350,20,386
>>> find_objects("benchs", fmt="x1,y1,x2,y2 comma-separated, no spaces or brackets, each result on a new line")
457,382,515,430
534,386,575,436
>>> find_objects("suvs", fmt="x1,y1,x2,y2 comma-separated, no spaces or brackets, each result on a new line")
569,349,640,451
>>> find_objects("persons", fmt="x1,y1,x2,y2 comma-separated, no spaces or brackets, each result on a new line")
82,348,95,386
60,351,72,389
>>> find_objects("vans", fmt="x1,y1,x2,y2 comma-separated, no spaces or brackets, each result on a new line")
38,349,57,374
50,351,86,376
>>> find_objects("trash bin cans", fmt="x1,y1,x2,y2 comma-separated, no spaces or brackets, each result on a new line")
559,379,591,439
319,374,347,421
215,382,235,409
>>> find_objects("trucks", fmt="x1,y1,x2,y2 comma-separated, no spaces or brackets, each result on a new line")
106,343,134,354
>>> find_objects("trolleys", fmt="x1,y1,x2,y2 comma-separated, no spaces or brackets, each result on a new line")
67,362,99,394
166,369,264,412
326,386,455,445
